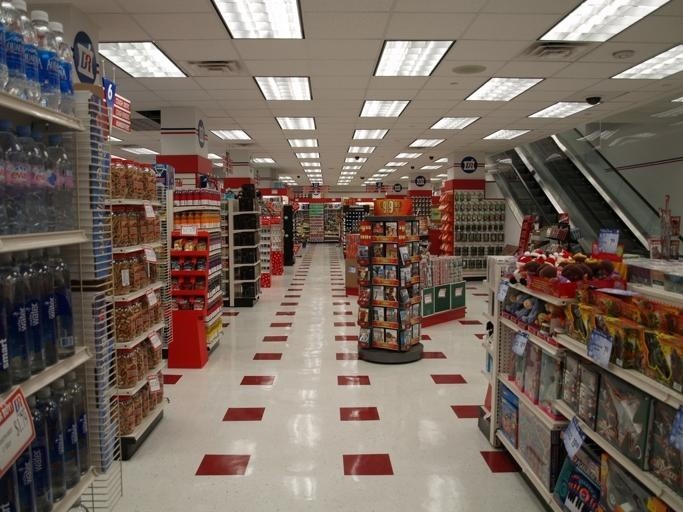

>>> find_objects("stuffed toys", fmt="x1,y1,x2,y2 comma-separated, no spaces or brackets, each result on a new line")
504,249,614,337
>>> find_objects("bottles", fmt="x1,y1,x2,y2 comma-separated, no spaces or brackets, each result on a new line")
117,332,163,389
112,206,161,247
115,287,164,344
120,372,164,434
205,314,223,344
113,250,159,296
109,158,158,200
173,209,221,230
1,255,76,395
0,370,92,512
0,118,80,234
209,237,222,252
208,271,221,299
204,295,224,323
209,250,223,275
173,188,222,207
0,0,77,116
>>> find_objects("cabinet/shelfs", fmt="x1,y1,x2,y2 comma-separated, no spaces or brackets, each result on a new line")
354,215,427,364
421,281,466,327
480,254,682,508
231,207,257,307
0,85,116,511
170,205,222,356
269,221,284,275
309,211,324,243
260,225,270,287
323,209,340,241
289,214,305,257
436,195,503,279
111,197,164,456
341,208,369,260
